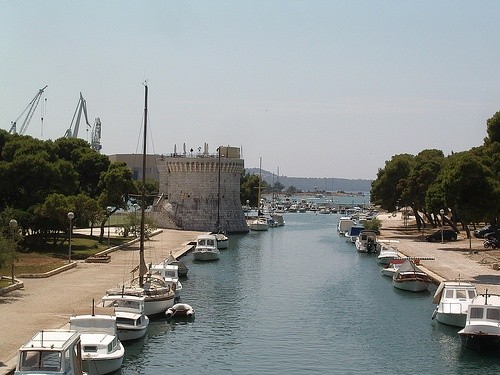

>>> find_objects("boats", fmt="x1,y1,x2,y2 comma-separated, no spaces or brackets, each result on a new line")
211,229,229,249
354,229,379,253
336,216,358,236
100,284,150,341
148,258,183,298
376,239,400,264
166,255,189,276
68,297,125,375
456,287,500,354
13,328,89,375
105,261,175,316
256,196,337,214
248,219,269,230
430,273,479,327
266,208,286,228
381,259,408,278
192,235,221,261
392,254,435,292
338,203,380,224
344,221,366,243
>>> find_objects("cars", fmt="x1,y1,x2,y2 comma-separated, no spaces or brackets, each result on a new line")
425,229,458,242
483,229,500,240
473,223,500,239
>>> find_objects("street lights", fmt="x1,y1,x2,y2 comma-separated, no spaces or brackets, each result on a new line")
133,204,138,239
67,212,74,264
246,199,250,222
9,219,17,283
106,206,112,249
